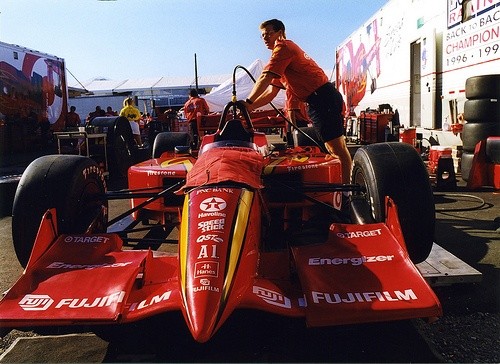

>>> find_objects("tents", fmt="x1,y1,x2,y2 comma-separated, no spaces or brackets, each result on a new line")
200,59,287,113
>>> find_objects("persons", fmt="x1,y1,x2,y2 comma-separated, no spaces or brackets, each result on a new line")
184,89,209,145
119,97,142,148
65,106,81,147
84,105,153,132
238,19,353,201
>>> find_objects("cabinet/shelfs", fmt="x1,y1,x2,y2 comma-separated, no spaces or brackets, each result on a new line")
360,114,392,143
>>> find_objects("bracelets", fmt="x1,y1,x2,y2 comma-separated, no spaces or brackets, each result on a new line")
246,98,252,105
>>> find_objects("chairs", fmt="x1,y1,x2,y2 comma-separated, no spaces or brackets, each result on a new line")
199,101,267,150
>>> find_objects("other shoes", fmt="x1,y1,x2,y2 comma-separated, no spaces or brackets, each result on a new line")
342,192,350,209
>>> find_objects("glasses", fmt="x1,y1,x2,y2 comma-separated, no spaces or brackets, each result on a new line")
260,31,276,40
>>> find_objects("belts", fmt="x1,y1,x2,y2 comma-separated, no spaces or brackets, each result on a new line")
307,83,331,104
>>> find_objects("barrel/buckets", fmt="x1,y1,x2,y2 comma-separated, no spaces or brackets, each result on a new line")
399,128,417,147
429,145,452,174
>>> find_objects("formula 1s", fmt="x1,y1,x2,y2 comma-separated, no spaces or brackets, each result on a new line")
1,99,436,345
